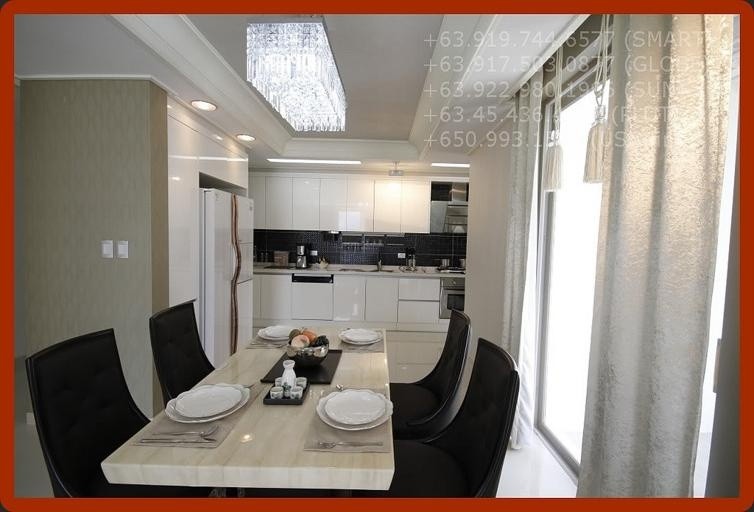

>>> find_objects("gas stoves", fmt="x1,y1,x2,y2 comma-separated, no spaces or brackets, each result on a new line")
437,265,465,274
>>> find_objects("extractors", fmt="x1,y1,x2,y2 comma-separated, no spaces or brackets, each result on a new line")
430,183,467,236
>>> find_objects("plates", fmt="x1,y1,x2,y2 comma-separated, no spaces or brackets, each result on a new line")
257,324,304,341
165,382,250,423
336,327,384,346
314,389,393,432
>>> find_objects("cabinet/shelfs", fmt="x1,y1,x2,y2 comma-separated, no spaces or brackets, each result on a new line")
250,265,440,329
373,175,431,236
320,174,373,236
264,172,321,233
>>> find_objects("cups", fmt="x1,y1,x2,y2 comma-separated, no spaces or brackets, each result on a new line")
271,385,282,396
289,384,301,399
274,377,282,385
296,377,307,388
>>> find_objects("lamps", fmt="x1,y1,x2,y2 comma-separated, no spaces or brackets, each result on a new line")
242,13,350,135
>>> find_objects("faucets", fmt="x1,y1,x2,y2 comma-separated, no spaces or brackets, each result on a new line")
377,260,382,270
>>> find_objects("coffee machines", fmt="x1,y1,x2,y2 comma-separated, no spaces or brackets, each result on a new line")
295,244,308,268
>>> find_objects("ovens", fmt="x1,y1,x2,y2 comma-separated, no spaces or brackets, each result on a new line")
439,278,464,319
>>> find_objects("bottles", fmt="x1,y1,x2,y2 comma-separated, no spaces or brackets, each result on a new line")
406,249,415,266
281,359,296,395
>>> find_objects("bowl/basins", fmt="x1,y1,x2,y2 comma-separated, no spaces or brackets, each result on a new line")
286,345,328,368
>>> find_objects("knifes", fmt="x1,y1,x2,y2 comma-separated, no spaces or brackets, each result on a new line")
137,437,217,445
246,344,285,349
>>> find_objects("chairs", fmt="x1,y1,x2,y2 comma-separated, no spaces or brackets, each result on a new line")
354,335,526,499
21,324,222,498
382,304,477,441
146,296,222,411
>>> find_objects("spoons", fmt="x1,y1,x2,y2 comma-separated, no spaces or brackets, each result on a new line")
314,441,383,450
152,424,219,438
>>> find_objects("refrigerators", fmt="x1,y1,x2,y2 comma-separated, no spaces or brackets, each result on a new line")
195,185,255,368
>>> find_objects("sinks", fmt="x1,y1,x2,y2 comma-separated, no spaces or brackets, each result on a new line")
338,268,394,273
